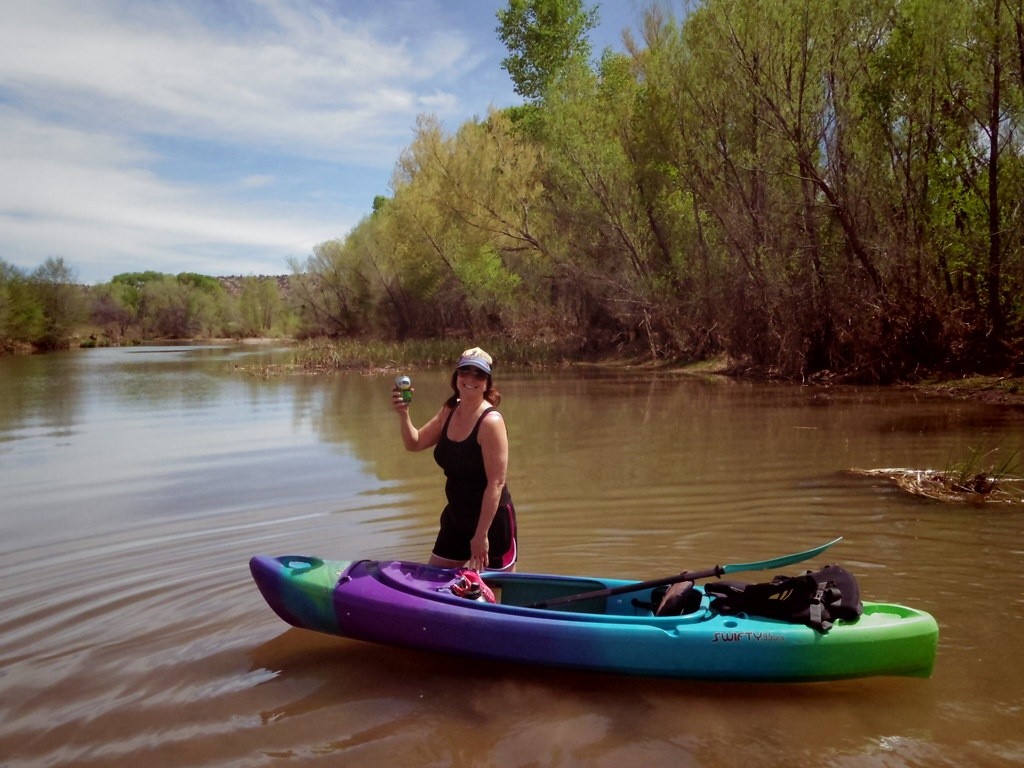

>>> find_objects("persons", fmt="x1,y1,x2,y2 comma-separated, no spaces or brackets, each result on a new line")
391,347,517,572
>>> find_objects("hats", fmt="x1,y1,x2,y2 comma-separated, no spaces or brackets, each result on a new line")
456,347,492,374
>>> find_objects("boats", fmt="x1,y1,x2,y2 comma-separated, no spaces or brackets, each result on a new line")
248,552,939,681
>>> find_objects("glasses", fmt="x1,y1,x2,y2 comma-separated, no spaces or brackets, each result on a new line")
459,366,489,381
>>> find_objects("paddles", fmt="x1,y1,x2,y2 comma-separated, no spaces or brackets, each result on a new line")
515,539,849,596
